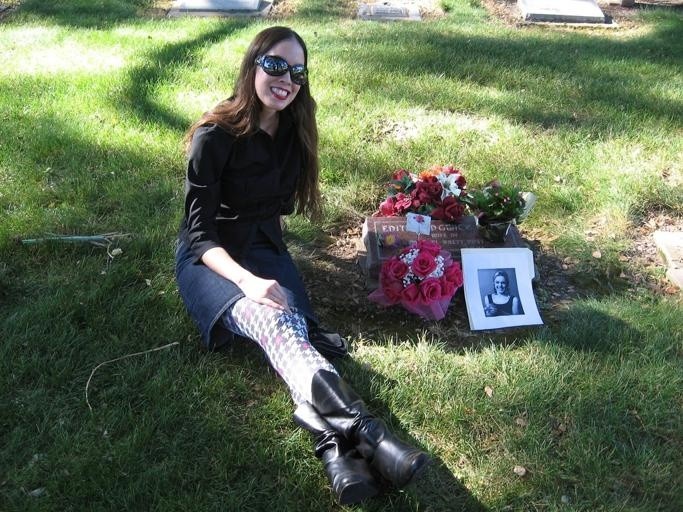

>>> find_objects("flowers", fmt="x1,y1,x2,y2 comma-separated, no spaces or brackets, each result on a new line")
372,164,469,224
465,178,533,246
367,242,461,319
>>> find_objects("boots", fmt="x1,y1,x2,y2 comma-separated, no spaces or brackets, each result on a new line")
311,369,429,489
293,400,383,504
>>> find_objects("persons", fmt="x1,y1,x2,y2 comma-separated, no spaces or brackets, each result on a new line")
174,27,427,501
483,270,519,316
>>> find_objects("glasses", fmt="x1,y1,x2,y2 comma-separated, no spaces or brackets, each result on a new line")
257,55,308,85
